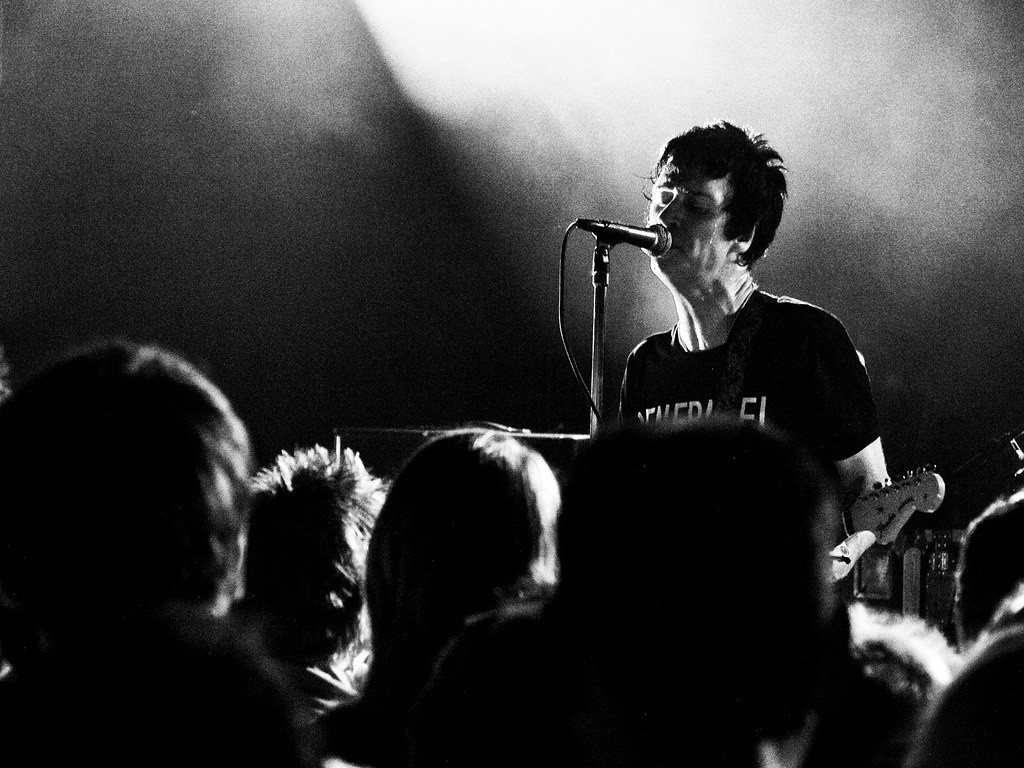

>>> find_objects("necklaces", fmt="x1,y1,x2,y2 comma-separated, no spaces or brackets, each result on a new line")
677,282,755,352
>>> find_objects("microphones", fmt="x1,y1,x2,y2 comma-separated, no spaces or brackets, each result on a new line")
575,218,673,258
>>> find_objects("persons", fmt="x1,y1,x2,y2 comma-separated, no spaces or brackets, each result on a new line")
222,432,392,724
405,415,1024,767
0,600,320,768
315,421,563,768
615,118,891,607
0,339,320,612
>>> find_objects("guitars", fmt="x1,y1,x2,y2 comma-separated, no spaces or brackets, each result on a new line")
833,464,945,558
